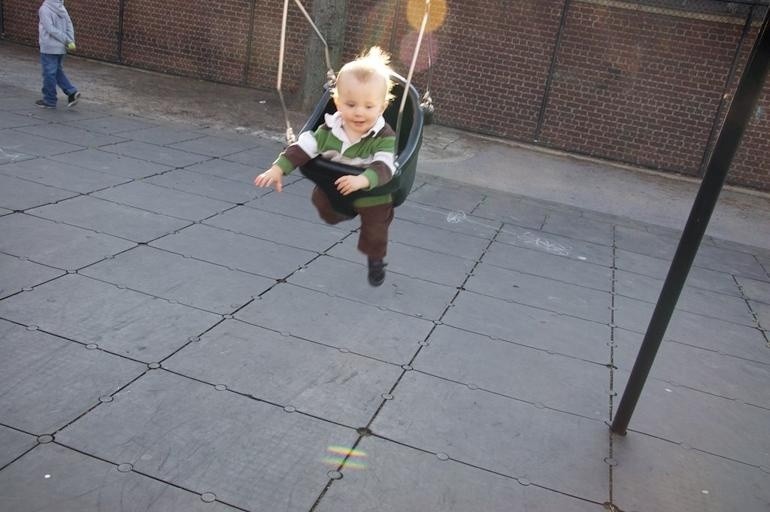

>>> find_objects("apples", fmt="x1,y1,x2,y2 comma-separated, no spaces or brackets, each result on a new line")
68,42,76,48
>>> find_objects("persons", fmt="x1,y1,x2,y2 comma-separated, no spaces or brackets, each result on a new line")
33,1,81,110
252,48,400,288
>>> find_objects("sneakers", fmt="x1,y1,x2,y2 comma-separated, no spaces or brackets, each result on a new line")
367,256,388,286
35,99,56,109
66,90,81,109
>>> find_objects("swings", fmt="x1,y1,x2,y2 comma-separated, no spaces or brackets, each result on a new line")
276,0,429,216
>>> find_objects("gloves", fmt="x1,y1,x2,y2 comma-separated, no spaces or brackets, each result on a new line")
67,41,77,52
